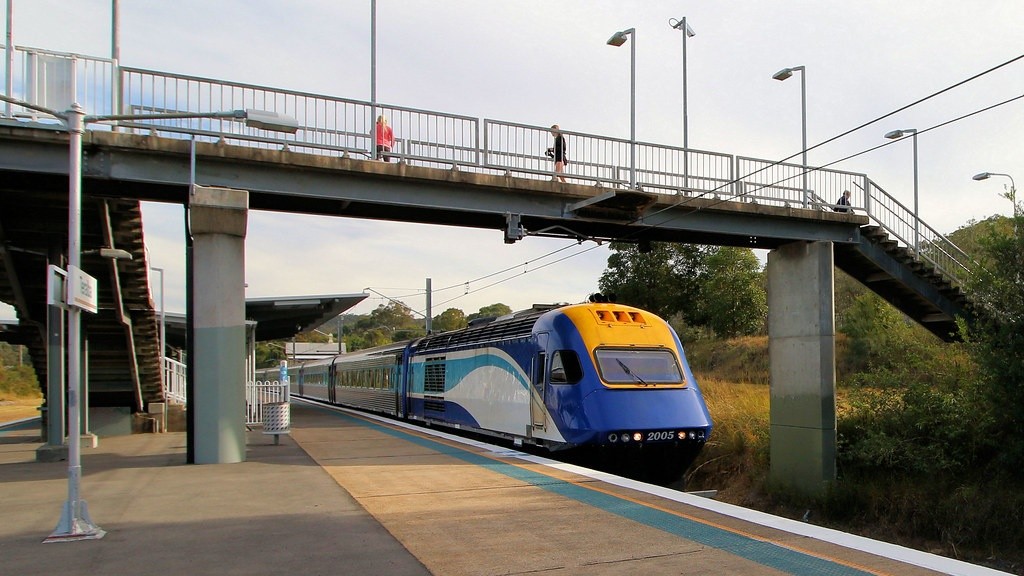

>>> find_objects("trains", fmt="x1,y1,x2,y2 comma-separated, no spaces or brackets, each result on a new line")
257,292,712,484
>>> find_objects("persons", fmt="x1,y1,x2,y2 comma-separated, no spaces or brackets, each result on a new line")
834,190,854,212
370,116,395,163
545,125,566,182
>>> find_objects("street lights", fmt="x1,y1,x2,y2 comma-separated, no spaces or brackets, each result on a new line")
43,100,299,543
36,247,132,464
883,127,921,261
973,172,1019,228
772,66,809,208
607,26,636,190
672,10,697,196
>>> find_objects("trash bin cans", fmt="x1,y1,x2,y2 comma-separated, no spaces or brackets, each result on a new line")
261,402,291,447
